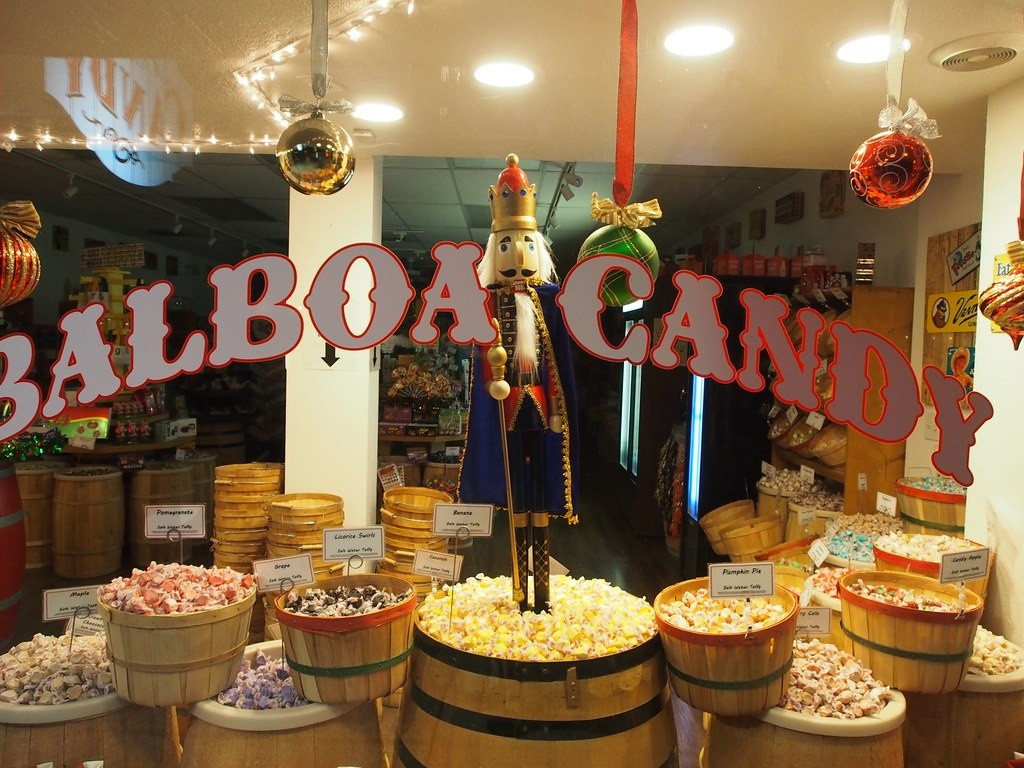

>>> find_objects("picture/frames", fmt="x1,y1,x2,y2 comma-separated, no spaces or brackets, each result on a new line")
51,224,179,276
675,170,846,260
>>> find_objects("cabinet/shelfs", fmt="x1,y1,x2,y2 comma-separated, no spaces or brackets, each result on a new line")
176,368,261,423
50,265,197,463
595,274,915,584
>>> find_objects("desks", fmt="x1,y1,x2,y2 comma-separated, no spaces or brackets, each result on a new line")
378,434,466,457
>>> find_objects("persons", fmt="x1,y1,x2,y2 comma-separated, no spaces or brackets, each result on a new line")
456,153,564,611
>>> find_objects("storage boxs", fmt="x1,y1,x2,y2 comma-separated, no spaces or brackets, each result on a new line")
383,354,415,383
407,427,436,436
379,425,407,436
406,441,460,460
222,391,253,415
155,394,197,442
680,255,833,288
382,402,413,423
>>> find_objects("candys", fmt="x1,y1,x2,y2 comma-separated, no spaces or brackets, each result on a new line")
386,363,452,399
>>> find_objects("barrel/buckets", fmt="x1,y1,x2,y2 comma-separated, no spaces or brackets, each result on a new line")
0,458,1024,768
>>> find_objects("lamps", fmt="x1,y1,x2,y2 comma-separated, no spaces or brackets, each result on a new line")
549,216,561,230
207,229,217,246
392,231,407,243
544,235,554,246
414,250,426,260
172,215,183,235
561,185,576,202
566,172,584,187
62,174,80,199
